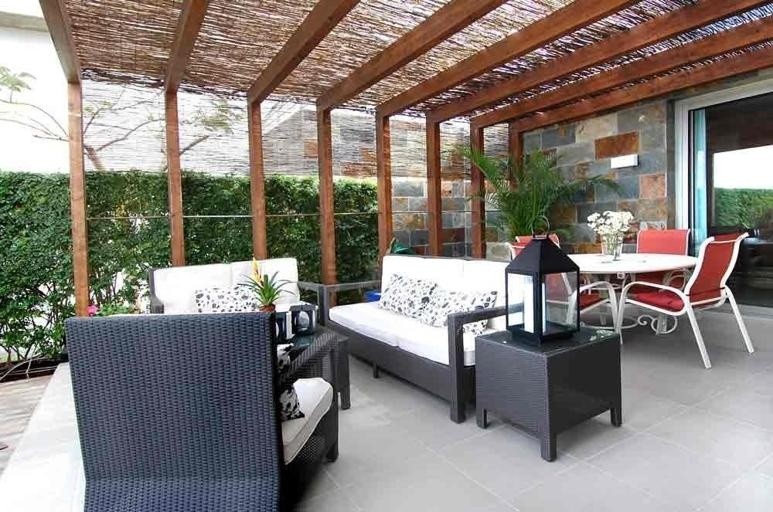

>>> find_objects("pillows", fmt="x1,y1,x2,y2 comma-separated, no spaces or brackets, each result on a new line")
378,273,497,337
193,288,260,314
277,343,305,421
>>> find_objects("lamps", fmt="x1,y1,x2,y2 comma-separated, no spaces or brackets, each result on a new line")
504,215,580,345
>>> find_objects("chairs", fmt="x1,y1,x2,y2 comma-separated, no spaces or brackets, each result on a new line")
622,229,691,331
64,311,339,512
505,241,623,347
616,233,755,369
516,234,586,295
148,256,323,326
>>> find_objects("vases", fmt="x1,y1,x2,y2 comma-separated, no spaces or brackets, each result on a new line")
600,234,623,261
259,305,276,313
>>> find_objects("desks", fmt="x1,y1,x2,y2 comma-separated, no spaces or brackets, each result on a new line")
567,253,694,341
299,315,351,410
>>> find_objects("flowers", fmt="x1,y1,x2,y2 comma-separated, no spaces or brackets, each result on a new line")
587,211,633,254
236,256,297,306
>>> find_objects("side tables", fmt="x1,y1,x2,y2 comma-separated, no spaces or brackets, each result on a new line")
476,327,622,461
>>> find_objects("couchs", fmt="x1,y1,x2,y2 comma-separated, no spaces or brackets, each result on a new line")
322,255,546,424
